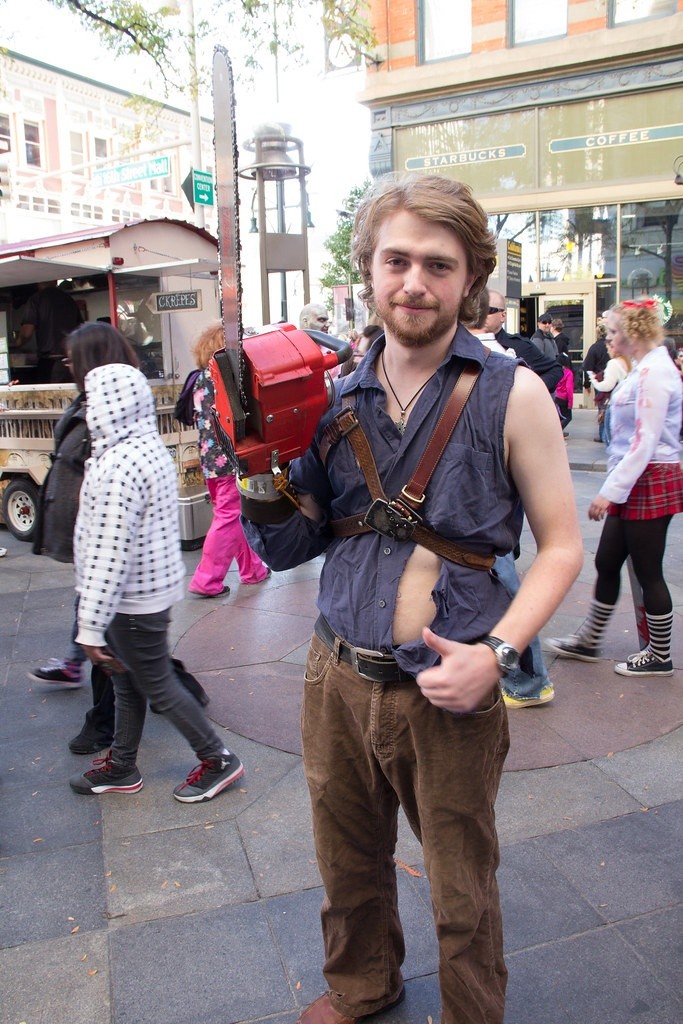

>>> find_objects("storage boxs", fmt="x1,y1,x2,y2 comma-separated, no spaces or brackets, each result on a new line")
180,485,215,552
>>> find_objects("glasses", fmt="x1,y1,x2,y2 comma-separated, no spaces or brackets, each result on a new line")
81,401,90,410
489,307,504,314
542,320,551,324
61,358,74,367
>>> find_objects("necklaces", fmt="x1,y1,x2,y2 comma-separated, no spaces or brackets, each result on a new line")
382,349,437,435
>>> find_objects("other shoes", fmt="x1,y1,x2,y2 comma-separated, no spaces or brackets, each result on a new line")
150,692,210,714
69,734,112,754
265,566,272,579
594,437,602,443
0,548,8,557
200,586,231,598
500,684,555,708
564,433,570,439
296,987,404,1024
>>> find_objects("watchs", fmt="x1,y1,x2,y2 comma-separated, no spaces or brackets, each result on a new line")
482,635,519,674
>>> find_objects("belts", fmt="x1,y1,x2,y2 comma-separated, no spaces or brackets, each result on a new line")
313,613,419,683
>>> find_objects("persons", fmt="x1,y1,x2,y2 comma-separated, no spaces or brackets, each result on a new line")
550,318,569,354
176,320,271,598
484,290,565,394
547,300,683,675
530,314,570,439
236,174,586,1024
299,301,384,382
27,321,245,803
553,352,575,430
467,288,554,710
581,324,629,443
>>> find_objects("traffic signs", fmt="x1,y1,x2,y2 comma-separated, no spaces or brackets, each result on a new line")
192,169,214,207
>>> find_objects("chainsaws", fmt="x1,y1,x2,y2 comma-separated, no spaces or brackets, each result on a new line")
209,44,354,481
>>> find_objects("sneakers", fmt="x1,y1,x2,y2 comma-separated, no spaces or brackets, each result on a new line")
614,651,674,677
173,751,244,803
545,634,602,662
27,658,82,687
71,751,144,795
